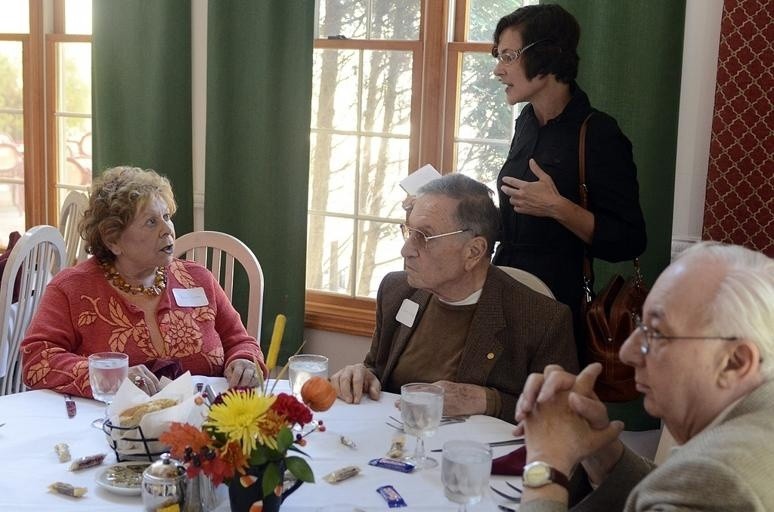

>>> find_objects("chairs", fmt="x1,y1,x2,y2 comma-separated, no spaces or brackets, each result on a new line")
495,262,578,417
170,230,266,346
0,191,91,397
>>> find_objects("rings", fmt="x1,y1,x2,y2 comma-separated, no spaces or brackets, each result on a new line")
134,376,145,389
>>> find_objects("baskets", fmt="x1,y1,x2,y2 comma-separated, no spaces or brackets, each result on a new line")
102,419,171,462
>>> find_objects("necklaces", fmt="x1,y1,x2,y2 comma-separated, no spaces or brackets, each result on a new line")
96,255,166,297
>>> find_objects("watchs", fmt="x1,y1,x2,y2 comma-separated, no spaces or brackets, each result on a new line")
521,460,571,493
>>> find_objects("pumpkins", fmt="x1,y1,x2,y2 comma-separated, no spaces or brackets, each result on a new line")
300,375,336,412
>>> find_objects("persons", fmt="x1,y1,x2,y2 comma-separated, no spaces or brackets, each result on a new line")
18,166,268,403
402,4,647,375
329,172,577,426
512,241,774,512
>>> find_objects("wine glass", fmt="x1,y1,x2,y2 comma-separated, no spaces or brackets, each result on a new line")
441,440,494,512
400,381,444,470
87,351,130,432
288,354,330,434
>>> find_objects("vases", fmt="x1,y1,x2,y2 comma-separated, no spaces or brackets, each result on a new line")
226,453,306,512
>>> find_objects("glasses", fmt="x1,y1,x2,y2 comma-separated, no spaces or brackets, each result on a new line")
492,39,549,66
399,223,472,252
629,308,762,363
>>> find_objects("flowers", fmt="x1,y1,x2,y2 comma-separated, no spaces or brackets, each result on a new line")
161,316,336,495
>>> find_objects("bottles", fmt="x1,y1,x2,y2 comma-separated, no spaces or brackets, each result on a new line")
142,446,218,511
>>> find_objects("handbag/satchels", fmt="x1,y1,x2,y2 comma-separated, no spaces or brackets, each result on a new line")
583,273,650,403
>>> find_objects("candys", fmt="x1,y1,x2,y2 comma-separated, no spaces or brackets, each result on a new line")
320,465,360,483
66,452,107,471
47,481,87,496
54,443,71,463
369,457,417,471
340,436,353,448
377,485,407,508
385,436,405,457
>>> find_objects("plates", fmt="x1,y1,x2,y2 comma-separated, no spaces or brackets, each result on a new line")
94,460,156,497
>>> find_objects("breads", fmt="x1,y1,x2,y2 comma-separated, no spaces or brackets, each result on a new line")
116,399,176,438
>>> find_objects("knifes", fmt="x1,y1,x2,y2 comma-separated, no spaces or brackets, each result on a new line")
431,438,526,453
